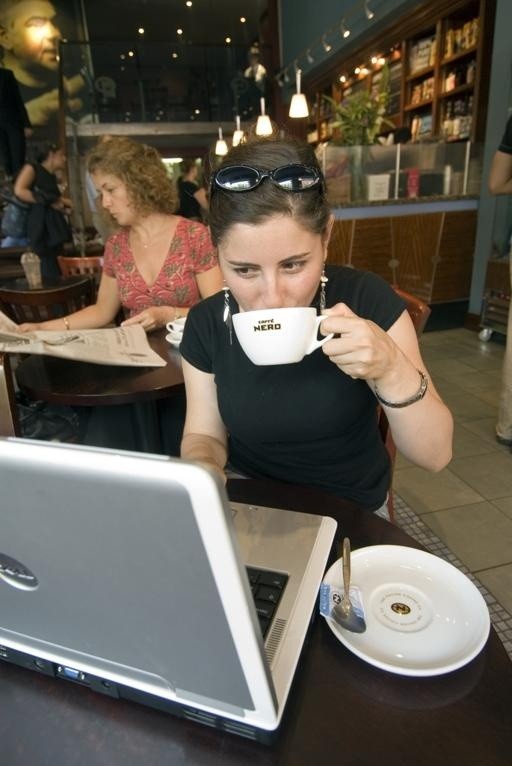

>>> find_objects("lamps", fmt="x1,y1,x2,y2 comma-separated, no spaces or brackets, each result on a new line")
214,127,228,156
232,114,245,147
256,97,273,136
289,68,310,118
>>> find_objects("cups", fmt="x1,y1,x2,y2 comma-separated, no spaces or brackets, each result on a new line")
232,308,336,367
21,252,42,289
165,319,187,338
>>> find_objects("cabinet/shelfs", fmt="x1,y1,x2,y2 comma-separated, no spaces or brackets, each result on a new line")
326,53,399,143
394,1,495,161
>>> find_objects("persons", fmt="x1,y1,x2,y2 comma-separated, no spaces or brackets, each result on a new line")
487,114,512,444
10,141,75,252
171,158,209,222
180,139,453,522
16,139,225,334
1,2,91,129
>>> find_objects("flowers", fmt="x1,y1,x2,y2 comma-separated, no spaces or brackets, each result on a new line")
320,62,393,205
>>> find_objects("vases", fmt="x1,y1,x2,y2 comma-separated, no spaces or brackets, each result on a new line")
347,145,363,202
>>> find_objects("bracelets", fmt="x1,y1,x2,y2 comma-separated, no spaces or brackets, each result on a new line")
369,371,427,407
61,317,69,329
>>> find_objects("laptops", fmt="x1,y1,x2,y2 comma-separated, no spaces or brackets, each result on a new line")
1,437,338,748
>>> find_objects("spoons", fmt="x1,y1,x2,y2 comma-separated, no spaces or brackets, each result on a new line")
332,537,368,636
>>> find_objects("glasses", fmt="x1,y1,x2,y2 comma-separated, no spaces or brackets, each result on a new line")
208,165,326,197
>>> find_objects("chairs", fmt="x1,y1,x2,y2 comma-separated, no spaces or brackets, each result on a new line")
1,275,93,322
58,255,104,282
0,245,31,280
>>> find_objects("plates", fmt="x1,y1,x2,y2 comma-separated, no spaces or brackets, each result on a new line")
316,544,492,677
166,332,183,345
